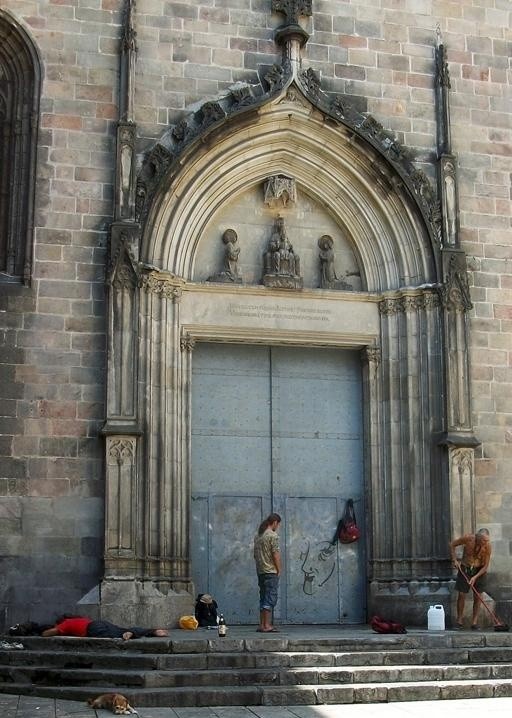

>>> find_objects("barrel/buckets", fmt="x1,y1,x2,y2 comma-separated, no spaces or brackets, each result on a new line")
427,604,445,631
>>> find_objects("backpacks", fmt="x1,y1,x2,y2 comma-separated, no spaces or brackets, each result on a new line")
195,594,218,627
337,498,360,544
371,615,407,634
6,621,53,636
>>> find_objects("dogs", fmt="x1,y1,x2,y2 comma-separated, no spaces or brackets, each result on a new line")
86,691,139,716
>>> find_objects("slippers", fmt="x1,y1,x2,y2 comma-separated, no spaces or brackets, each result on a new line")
456,623,464,628
471,624,480,630
256,627,280,633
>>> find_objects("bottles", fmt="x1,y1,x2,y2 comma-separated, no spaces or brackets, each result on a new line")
217,614,228,637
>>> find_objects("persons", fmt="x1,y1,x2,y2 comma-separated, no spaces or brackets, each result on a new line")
254,512,281,633
449,528,492,629
41,614,169,640
318,235,335,286
269,225,295,276
222,229,241,276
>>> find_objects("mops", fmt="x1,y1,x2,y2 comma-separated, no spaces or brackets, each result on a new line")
455,560,512,632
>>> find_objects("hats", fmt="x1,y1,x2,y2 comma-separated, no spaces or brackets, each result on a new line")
200,595,212,604
